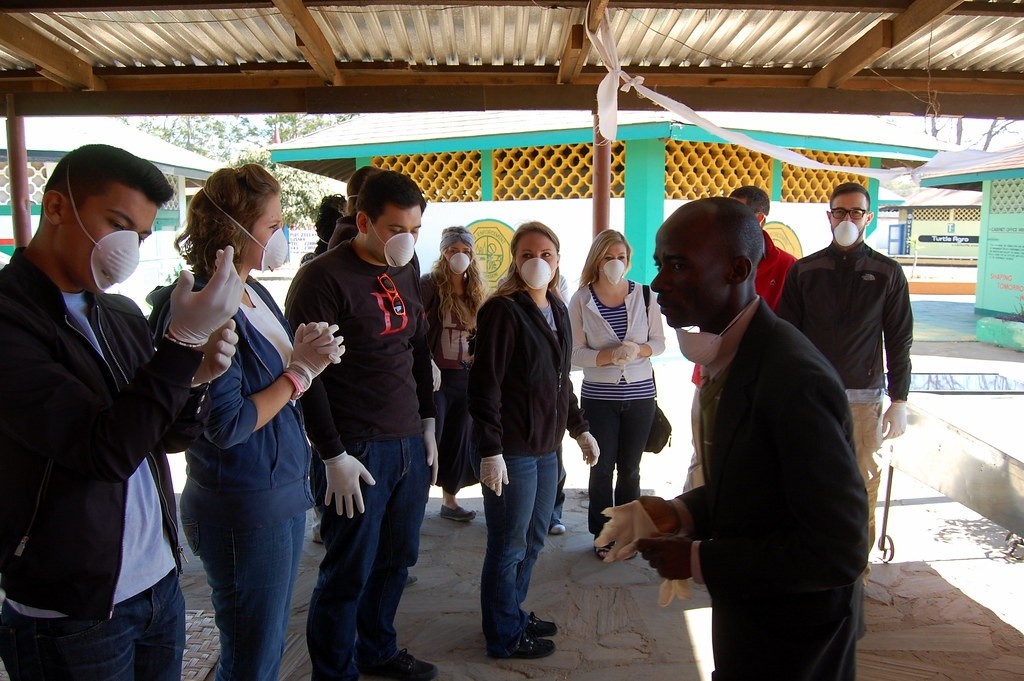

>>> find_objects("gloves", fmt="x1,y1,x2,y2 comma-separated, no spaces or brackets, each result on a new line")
189,318,238,388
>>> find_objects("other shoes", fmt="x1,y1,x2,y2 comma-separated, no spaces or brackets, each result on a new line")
593,538,615,560
548,521,566,534
405,576,418,587
526,611,557,636
510,632,556,659
441,504,476,522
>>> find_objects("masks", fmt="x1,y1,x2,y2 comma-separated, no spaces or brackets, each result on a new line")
383,232,415,267
91,231,140,291
521,258,552,290
834,220,859,247
449,252,470,275
261,227,288,274
675,326,724,366
603,259,625,286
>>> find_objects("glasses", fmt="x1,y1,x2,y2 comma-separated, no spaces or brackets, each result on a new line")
831,207,867,220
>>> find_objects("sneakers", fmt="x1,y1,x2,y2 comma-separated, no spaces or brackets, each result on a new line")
357,648,438,681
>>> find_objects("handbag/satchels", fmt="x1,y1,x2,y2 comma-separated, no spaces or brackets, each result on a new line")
643,405,672,454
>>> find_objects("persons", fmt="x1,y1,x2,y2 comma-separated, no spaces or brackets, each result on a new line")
145,164,347,681
0,144,244,681
285,166,914,681
